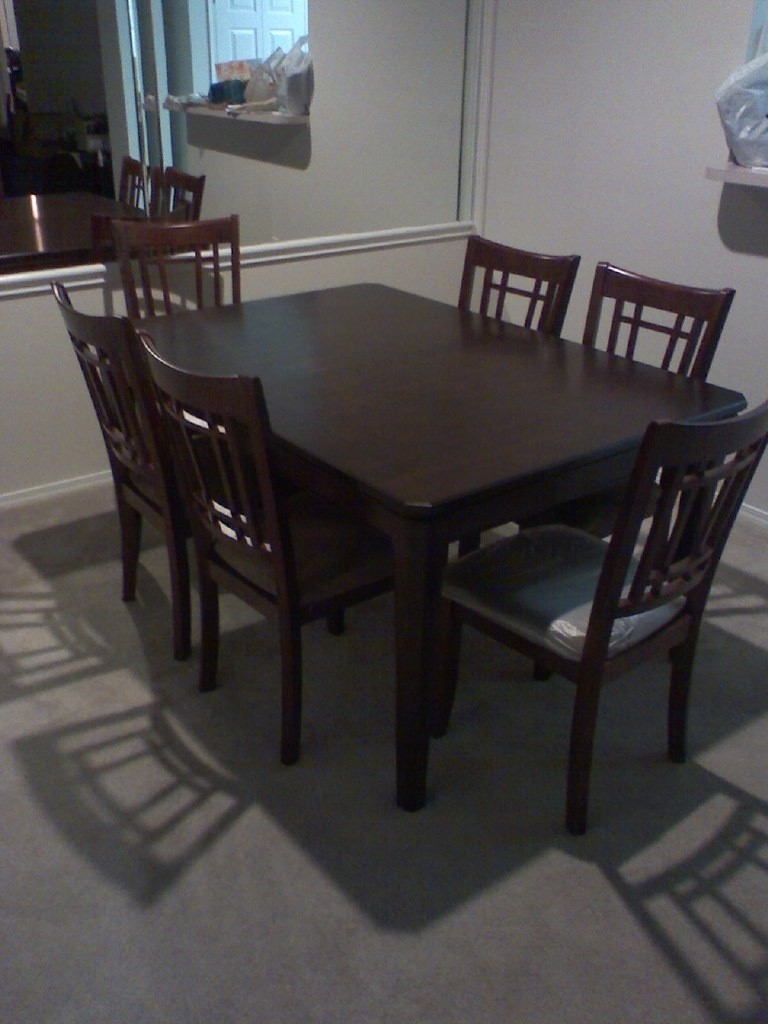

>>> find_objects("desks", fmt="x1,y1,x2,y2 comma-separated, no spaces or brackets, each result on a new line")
133,283,746,811
0,193,151,274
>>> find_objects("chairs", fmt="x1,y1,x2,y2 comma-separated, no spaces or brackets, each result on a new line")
116,155,163,221
429,399,767,835
135,325,398,766
460,233,581,337
51,282,255,659
162,164,205,221
114,215,245,321
458,262,738,561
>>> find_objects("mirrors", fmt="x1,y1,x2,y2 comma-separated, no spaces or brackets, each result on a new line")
0,1,501,296
